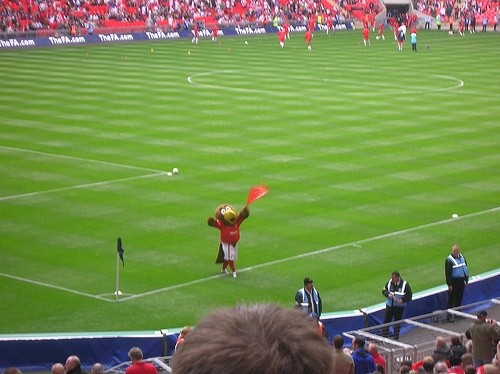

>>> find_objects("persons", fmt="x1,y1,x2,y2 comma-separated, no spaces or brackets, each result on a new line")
0,0,500,51
125,347,159,374
2,367,21,374
52,355,106,374
381,271,412,341
173,304,500,374
295,277,322,317
445,244,469,323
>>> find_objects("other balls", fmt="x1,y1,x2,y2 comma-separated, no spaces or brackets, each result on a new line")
173,168,179,174
452,213,459,219
114,290,122,295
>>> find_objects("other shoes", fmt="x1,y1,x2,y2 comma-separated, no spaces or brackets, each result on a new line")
454,314,461,318
446,318,454,322
221,269,229,275
233,272,237,278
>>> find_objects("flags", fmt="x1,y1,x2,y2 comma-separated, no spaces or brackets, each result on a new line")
116,236,125,267
243,184,269,210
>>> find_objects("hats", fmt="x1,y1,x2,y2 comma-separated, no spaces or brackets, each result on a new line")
304,277,314,285
180,326,192,337
477,311,487,318
128,347,143,359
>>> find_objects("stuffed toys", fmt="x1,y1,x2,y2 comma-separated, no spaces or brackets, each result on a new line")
207,202,249,278
